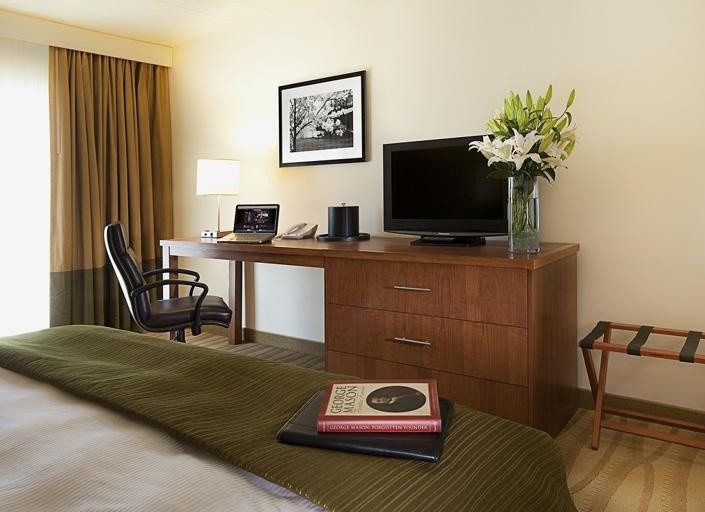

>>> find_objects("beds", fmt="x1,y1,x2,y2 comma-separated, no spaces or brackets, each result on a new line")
0,323,577,511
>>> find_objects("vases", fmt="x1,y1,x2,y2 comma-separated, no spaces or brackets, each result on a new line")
506,174,541,255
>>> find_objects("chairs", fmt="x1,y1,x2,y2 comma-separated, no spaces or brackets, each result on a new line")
102,220,233,342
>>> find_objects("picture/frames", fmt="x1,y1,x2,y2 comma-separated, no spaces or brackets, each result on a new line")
277,69,366,167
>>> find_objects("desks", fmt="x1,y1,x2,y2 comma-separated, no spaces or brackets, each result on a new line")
156,232,582,440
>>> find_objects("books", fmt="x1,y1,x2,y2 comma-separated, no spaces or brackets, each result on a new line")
316,379,442,433
276,387,455,463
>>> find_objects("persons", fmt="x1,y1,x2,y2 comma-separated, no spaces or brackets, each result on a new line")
370,388,421,411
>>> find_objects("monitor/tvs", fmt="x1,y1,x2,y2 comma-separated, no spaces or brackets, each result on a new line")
382,133,508,247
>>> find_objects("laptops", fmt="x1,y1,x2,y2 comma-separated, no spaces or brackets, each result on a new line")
217,204,280,244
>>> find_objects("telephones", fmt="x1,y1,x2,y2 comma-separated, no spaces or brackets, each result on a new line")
281,223,319,240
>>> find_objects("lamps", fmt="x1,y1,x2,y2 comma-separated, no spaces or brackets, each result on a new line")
195,158,245,240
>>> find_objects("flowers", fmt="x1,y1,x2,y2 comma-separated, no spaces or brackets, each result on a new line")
467,83,581,236
